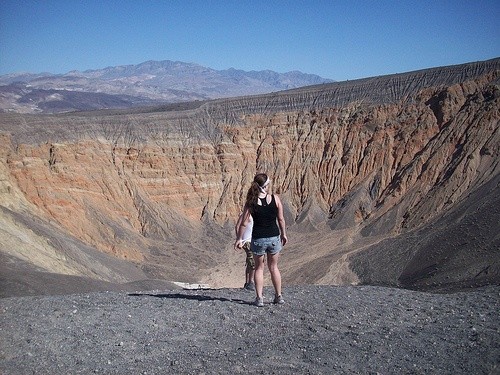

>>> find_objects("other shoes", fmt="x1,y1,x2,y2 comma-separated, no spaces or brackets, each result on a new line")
273,293,284,304
254,296,264,306
244,282,249,289
246,283,255,291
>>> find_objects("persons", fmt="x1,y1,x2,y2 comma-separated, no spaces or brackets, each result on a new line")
234,174,287,306
235,212,256,291
